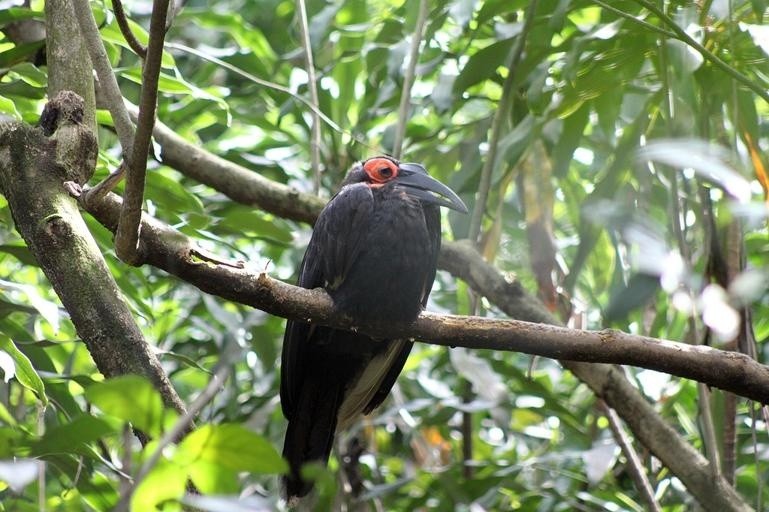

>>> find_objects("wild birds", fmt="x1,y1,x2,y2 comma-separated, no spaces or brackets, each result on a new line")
279,154,469,504
